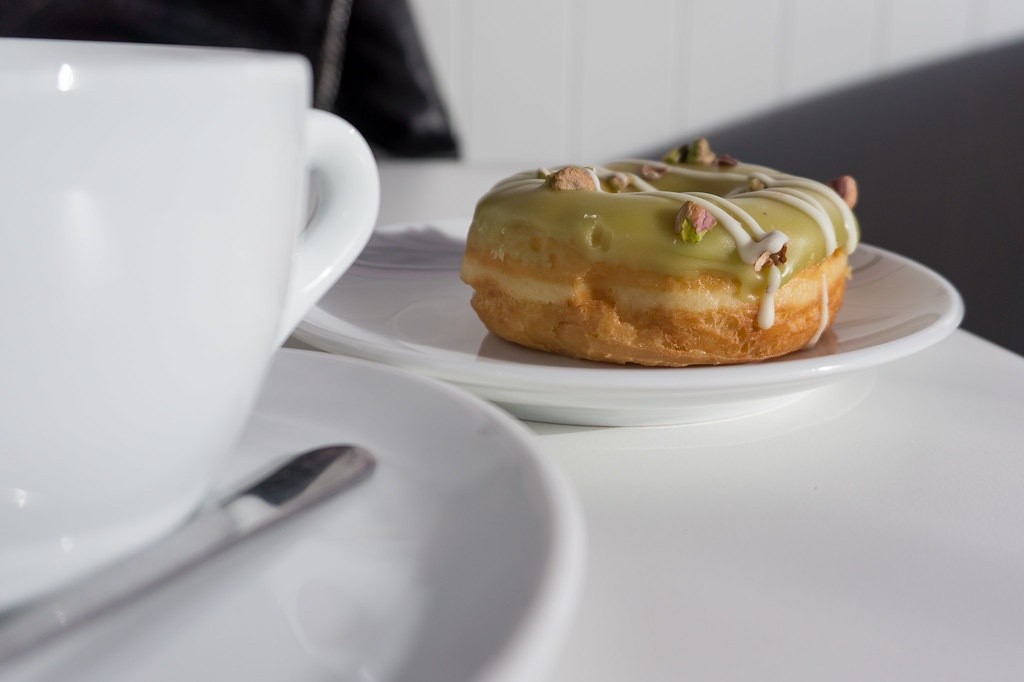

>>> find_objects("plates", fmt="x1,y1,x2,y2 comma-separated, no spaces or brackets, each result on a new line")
0,348,587,682
291,220,964,427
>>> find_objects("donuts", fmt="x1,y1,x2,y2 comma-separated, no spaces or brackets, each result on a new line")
463,141,859,366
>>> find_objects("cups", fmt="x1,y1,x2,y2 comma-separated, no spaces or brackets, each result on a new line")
0,38,381,610
